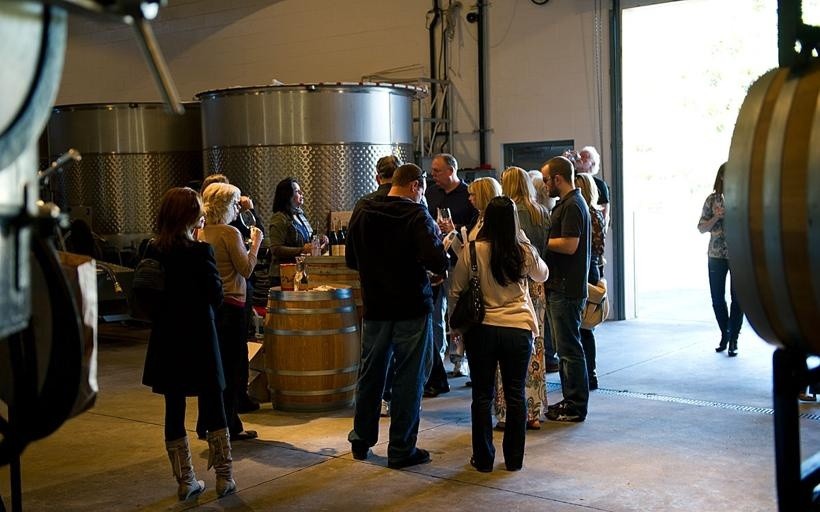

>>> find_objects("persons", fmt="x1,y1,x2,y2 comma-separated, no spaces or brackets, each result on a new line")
136,174,265,500
697,162,744,356
345,145,610,473
268,177,314,287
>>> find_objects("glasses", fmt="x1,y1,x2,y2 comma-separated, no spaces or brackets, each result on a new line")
543,176,551,183
410,170,428,182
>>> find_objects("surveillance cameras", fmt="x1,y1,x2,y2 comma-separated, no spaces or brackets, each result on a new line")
465,10,479,24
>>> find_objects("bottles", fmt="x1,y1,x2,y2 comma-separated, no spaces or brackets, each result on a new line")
311,235,321,256
329,220,348,257
294,257,308,291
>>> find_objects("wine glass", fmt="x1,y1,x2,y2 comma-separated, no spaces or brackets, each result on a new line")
715,193,726,219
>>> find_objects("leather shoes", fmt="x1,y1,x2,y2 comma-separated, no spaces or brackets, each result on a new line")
728,341,743,356
712,340,729,352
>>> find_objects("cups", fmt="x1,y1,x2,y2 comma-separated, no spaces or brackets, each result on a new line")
239,209,256,230
440,207,452,224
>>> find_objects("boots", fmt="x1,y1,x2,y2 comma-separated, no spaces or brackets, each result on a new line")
205,426,236,496
165,434,205,501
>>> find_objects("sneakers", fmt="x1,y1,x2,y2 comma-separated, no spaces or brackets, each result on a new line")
230,430,257,441
423,384,450,397
352,443,369,459
452,358,469,377
470,398,586,472
380,399,389,416
237,397,260,414
387,448,429,469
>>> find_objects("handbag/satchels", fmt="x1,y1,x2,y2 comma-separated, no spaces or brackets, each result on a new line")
126,237,166,323
449,240,485,330
29,223,99,419
580,278,609,330
